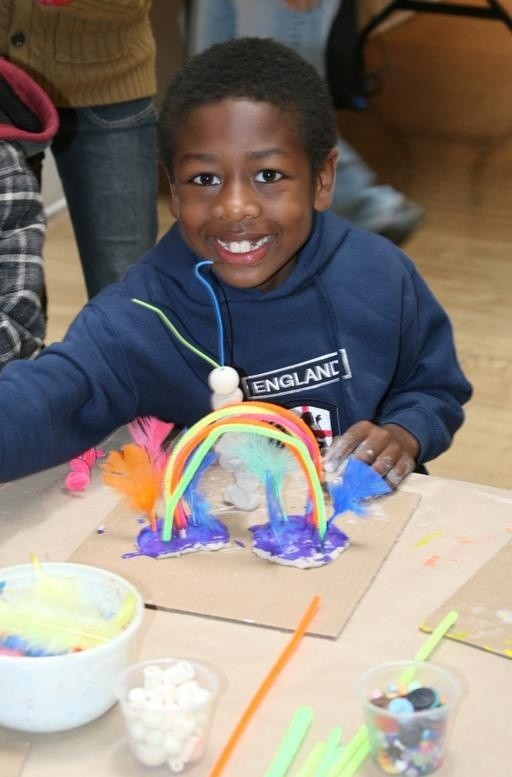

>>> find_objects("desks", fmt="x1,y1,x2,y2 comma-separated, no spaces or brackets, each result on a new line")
2,415,511,776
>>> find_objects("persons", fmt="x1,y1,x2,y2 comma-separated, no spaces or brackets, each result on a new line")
190,1,419,252
0,1,163,304
0,55,64,368
2,32,472,500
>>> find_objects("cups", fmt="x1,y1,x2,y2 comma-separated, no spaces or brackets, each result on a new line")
356,658,466,776
113,656,225,772
